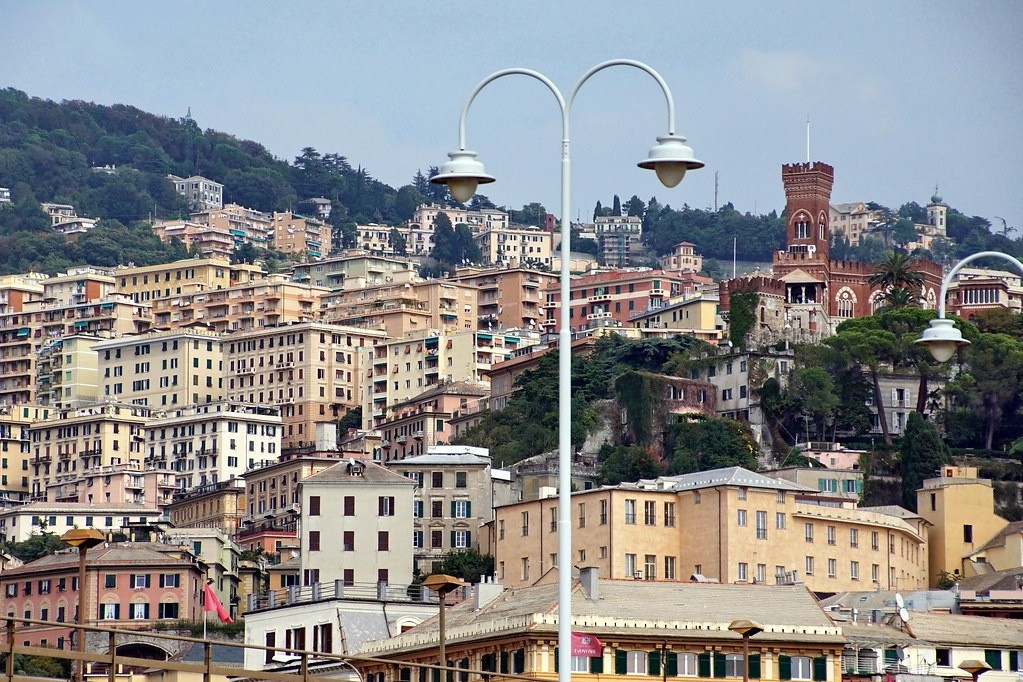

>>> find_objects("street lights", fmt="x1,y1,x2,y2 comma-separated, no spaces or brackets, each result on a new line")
421,574,465,682
728,619,765,682
958,659,992,682
59,529,107,682
430,56,706,682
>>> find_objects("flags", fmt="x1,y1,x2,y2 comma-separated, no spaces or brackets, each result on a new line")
204,583,233,624
